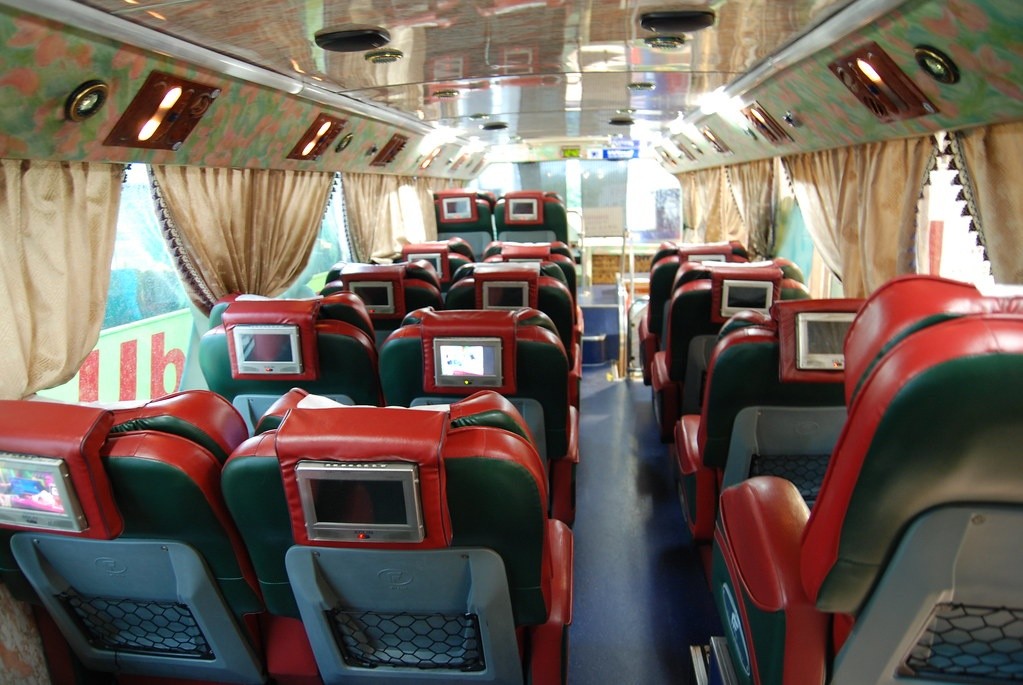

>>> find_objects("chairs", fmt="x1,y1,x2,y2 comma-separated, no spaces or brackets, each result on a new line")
0,190,1023,685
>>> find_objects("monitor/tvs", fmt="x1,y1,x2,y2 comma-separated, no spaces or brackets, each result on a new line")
0,197,539,545
691,254,857,369
